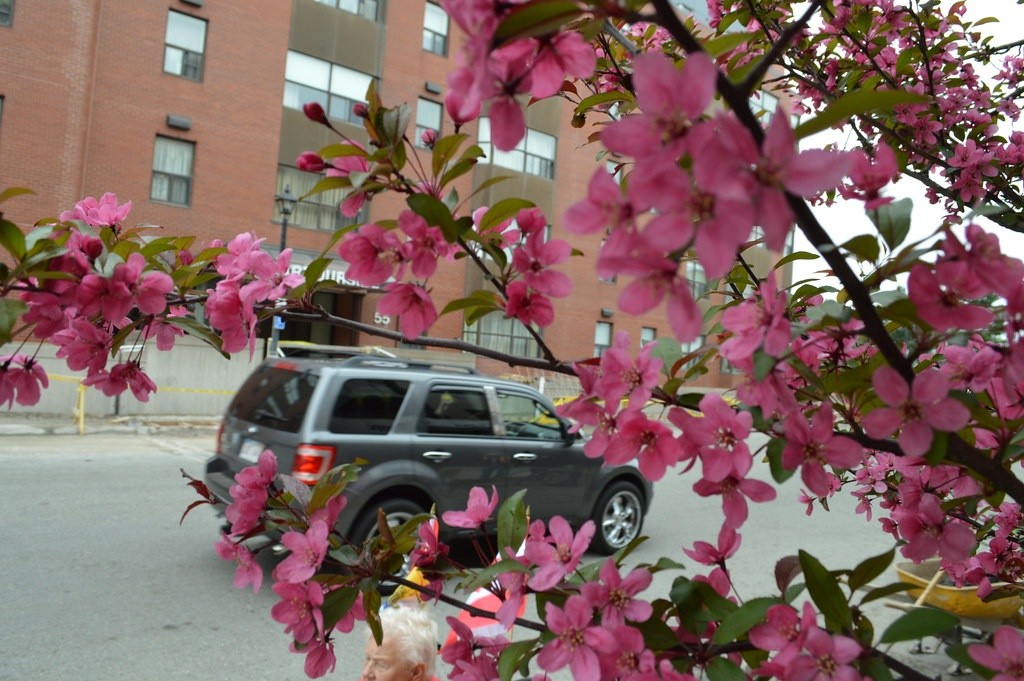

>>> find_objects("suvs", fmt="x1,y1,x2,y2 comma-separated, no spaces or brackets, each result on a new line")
202,348,653,595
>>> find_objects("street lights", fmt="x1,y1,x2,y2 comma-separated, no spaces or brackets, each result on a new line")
274,183,298,253
